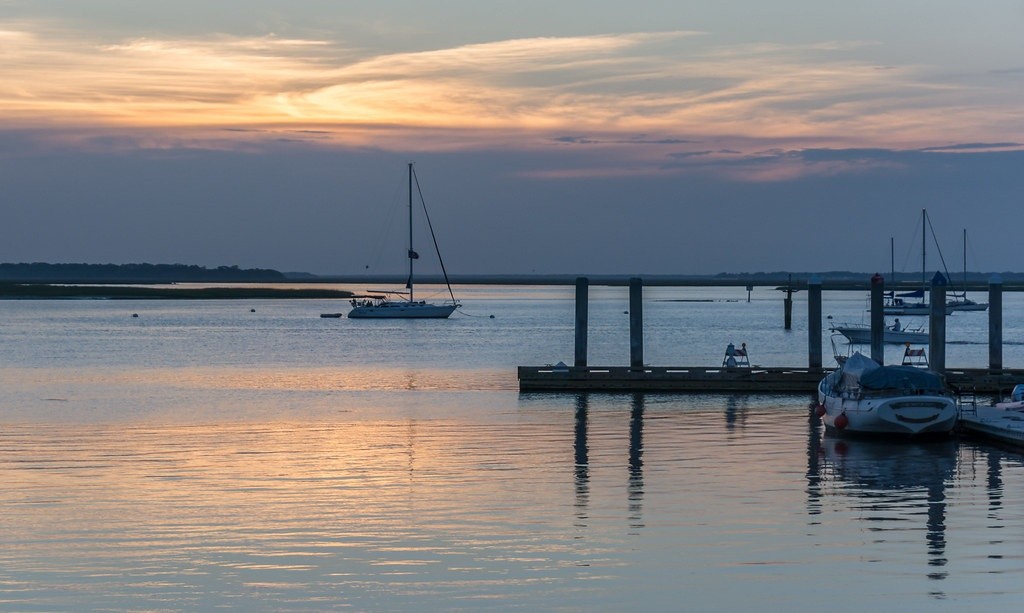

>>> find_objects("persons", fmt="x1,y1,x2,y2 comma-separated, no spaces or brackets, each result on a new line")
889,318,900,332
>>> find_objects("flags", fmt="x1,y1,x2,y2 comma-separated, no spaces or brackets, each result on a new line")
408,249,419,260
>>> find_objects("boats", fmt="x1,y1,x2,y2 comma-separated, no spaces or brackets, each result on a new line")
818,351,962,441
954,383,1024,450
829,318,928,345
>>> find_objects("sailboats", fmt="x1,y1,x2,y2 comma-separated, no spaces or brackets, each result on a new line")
946,227,991,312
866,207,962,315
348,161,461,319
